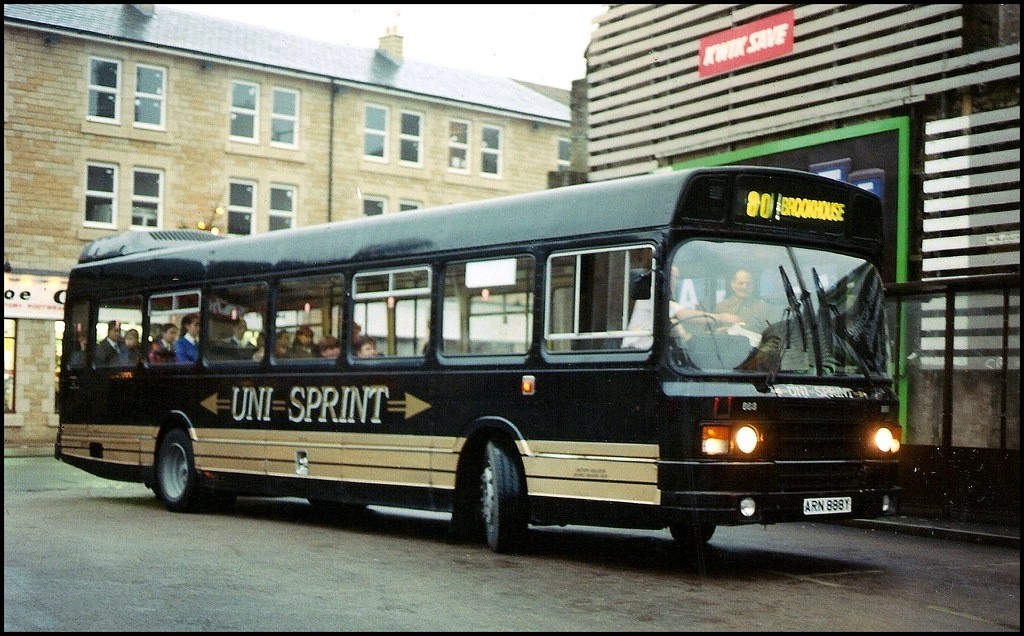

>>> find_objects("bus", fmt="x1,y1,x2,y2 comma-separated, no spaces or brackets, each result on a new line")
53,164,902,553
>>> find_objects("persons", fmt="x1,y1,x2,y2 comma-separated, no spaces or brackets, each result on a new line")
274,330,292,357
319,336,341,357
714,266,773,336
351,322,362,355
621,263,741,349
354,337,378,358
76,310,265,370
292,324,321,358
